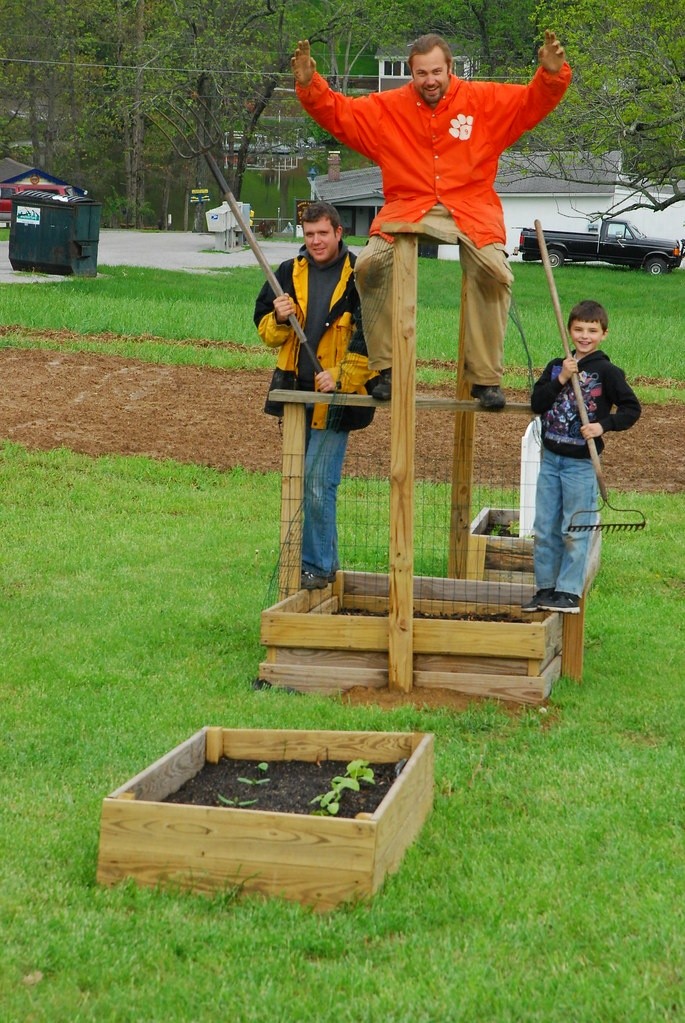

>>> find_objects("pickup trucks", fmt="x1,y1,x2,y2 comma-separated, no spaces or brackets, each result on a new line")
518,220,684,275
0,183,74,223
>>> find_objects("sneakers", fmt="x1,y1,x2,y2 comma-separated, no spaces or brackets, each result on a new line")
372,368,393,401
538,589,582,615
521,588,557,612
470,381,505,407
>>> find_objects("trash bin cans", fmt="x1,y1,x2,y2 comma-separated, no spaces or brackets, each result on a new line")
8,189,103,278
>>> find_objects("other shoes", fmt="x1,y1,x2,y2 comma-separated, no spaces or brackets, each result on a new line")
299,569,337,590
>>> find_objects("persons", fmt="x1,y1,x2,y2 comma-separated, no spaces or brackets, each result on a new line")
253,201,376,590
291,29,573,410
521,301,642,615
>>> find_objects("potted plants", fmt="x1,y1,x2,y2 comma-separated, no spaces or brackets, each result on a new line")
469,507,601,596
95,725,435,911
